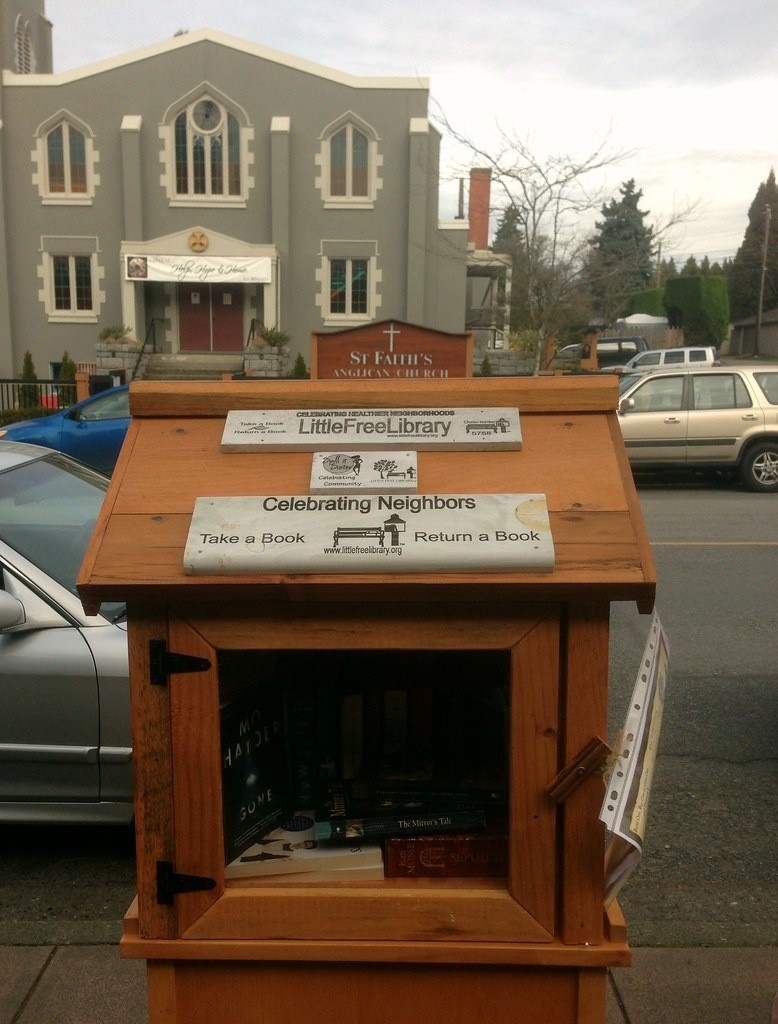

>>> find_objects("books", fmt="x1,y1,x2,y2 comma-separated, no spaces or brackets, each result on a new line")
220,676,509,884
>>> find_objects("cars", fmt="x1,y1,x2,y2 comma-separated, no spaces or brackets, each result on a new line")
617,365,778,493
0,383,132,473
0,438,135,827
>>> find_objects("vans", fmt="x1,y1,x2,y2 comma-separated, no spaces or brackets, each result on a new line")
623,346,719,372
558,337,649,367
